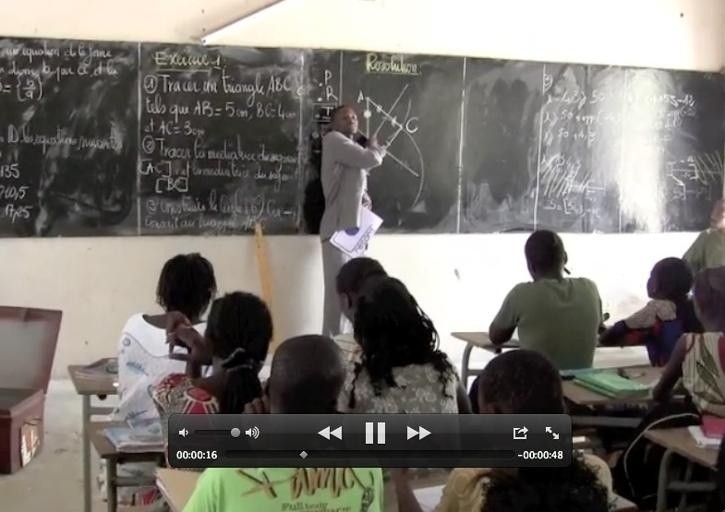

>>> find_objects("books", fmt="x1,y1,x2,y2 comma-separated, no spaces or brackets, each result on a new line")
75,358,118,381
330,204,383,260
102,416,165,453
573,371,649,403
685,414,724,450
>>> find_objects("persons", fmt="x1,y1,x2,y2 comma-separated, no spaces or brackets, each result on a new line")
106,252,216,422
469,229,602,414
478,447,618,512
431,349,614,512
136,258,474,512
320,106,383,339
599,196,724,425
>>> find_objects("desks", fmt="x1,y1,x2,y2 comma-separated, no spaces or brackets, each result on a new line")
69,361,122,511
386,472,637,512
85,420,168,511
644,426,718,511
449,330,519,395
555,363,684,456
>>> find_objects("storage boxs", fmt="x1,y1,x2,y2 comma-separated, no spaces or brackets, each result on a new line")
0,305,64,479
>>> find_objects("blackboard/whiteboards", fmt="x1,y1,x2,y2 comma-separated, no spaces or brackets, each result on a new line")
0,36,725,238
